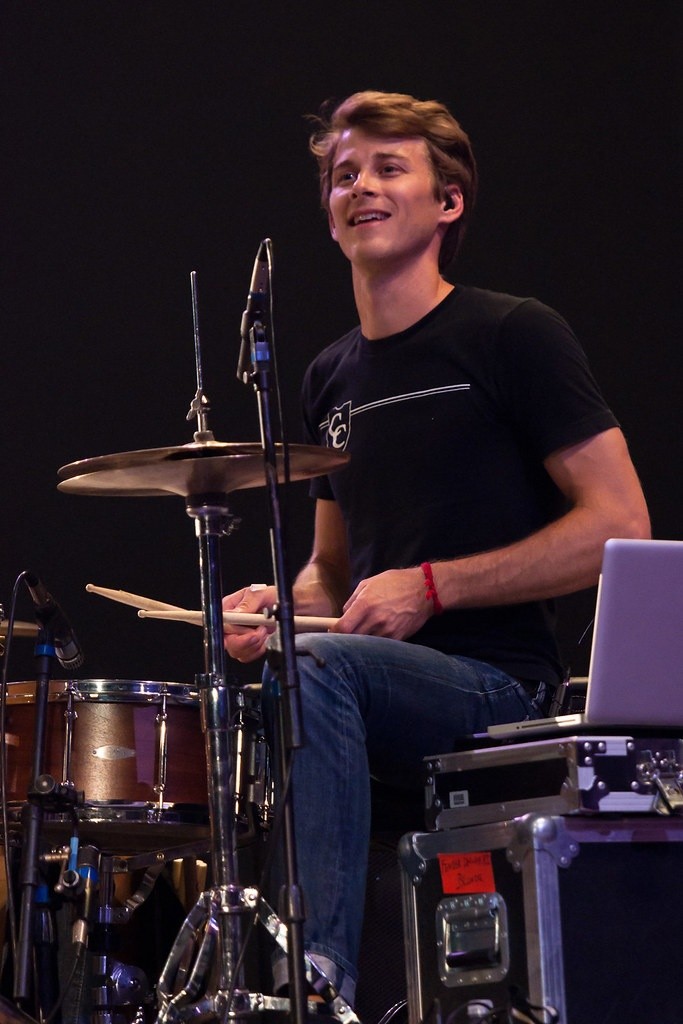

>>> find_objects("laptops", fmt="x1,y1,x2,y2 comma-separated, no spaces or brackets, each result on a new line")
486,538,682,739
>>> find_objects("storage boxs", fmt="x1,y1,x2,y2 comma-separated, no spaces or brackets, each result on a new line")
420,734,683,833
400,814,683,1024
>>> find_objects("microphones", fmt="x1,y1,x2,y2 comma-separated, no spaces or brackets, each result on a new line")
70,844,103,958
23,570,84,670
237,241,269,382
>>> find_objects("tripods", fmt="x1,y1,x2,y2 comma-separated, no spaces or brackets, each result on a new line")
151,494,364,1024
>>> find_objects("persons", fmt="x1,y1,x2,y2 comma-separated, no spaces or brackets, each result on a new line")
224,89,654,1008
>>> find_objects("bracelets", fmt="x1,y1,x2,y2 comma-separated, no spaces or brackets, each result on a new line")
420,561,446,613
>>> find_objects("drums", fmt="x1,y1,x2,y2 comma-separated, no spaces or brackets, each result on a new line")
0,678,237,854
72,853,209,975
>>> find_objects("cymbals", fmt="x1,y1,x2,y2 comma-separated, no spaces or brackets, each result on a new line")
56,438,352,497
0,618,40,637
